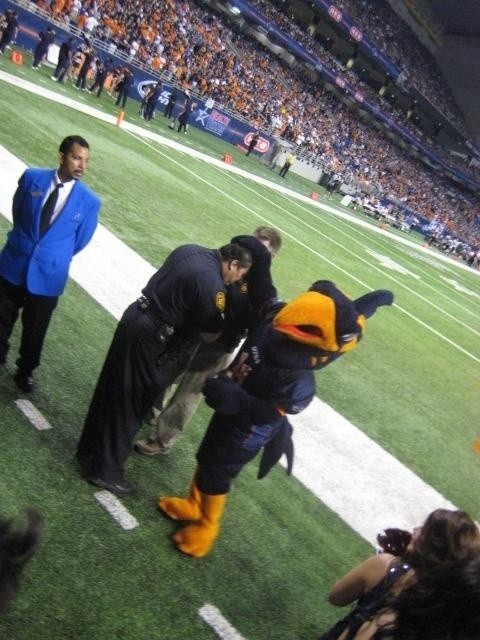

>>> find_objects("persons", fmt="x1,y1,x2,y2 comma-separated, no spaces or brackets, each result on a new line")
132,224,286,462
1,131,104,395
0,1,480,270
71,238,255,497
312,558,479,640
323,506,480,606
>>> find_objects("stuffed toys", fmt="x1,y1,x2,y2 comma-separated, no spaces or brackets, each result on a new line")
156,232,394,559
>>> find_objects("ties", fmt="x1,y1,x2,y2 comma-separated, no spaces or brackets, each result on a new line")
40,185,62,233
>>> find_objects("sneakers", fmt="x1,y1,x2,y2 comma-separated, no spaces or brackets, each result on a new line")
77,447,135,495
136,436,165,456
14,369,33,391
